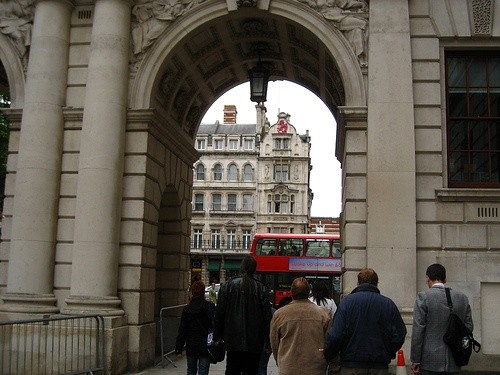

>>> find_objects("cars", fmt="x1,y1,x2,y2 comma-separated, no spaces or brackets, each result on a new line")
205,284,221,302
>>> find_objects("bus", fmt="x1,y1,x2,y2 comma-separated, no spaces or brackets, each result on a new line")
246,231,339,308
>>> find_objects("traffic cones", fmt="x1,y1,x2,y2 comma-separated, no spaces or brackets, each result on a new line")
396,349,408,375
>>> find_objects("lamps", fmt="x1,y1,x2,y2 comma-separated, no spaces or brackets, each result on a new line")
248,49,270,102
